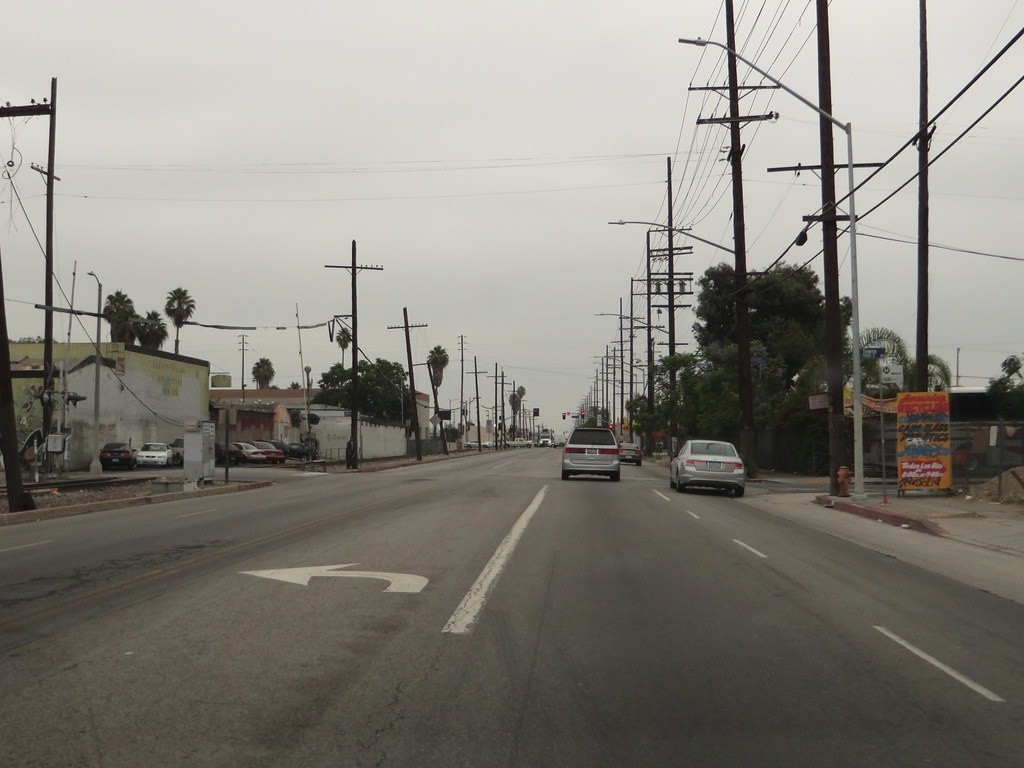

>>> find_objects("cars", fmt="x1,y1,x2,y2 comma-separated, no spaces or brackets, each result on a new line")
464,440,501,448
214,439,290,464
671,440,745,497
99,437,184,471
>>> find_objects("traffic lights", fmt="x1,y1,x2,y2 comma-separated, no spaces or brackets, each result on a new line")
609,424,613,429
562,413,566,419
618,443,643,466
581,411,585,419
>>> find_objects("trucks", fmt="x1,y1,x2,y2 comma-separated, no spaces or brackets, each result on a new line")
539,429,554,447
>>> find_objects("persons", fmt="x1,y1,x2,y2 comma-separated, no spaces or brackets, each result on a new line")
347,436,355,469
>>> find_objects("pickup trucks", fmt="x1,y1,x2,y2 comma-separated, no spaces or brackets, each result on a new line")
505,438,534,448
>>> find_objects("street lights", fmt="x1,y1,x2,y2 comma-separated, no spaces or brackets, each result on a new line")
86,271,102,474
678,37,867,500
594,219,757,480
